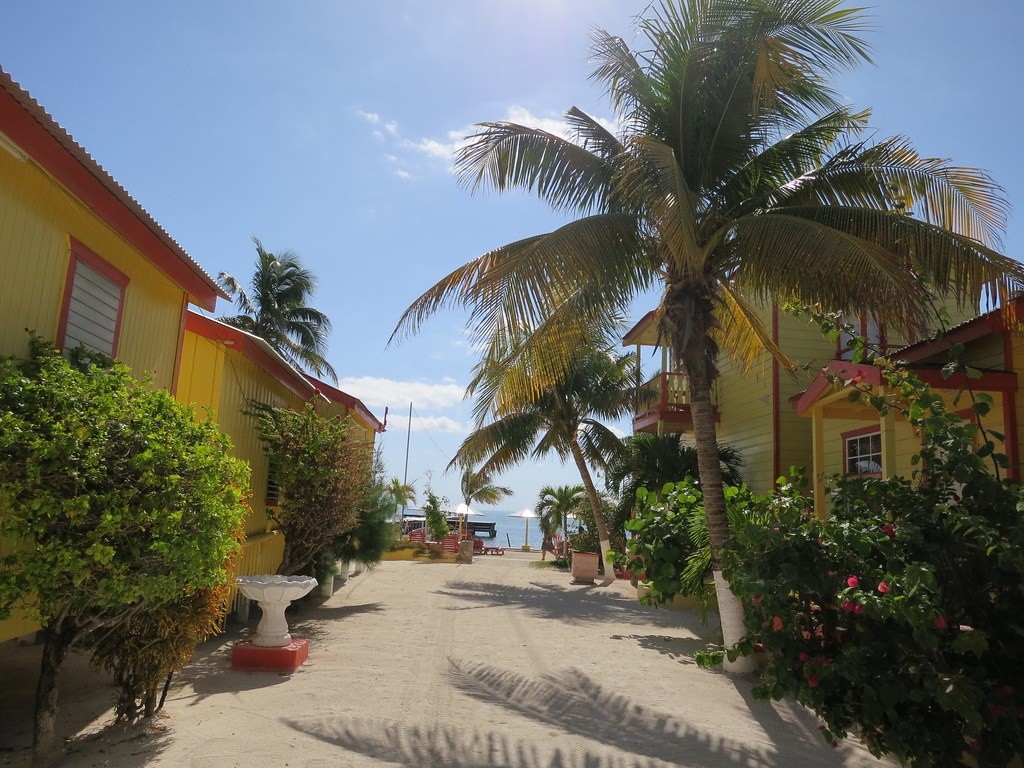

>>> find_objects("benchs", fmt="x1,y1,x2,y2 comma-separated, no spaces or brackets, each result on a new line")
408,529,505,555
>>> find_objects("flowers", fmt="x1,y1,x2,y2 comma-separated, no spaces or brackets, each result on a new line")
751,523,1017,752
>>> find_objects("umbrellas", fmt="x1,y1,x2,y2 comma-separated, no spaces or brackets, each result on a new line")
441,503,485,543
507,507,542,546
408,502,435,531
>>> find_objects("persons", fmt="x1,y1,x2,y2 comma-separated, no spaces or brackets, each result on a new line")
540,533,560,561
